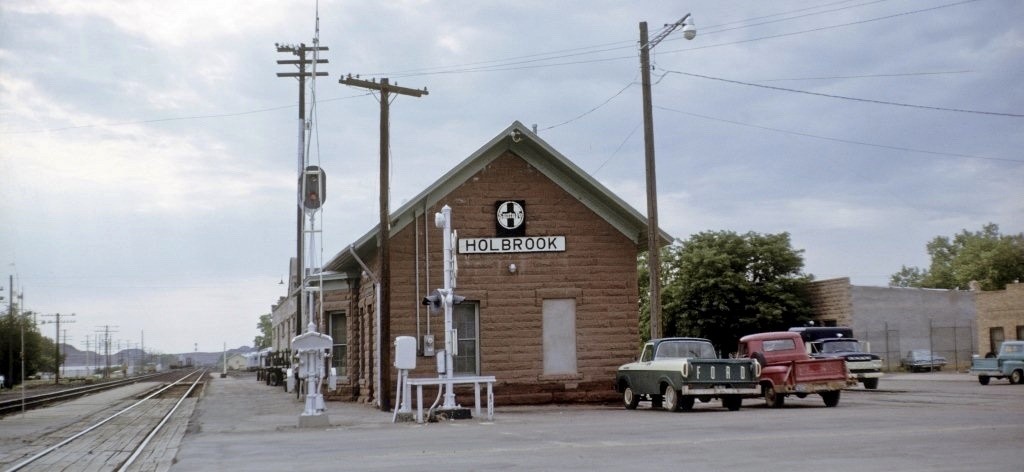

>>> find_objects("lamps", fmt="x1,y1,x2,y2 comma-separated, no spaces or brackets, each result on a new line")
362,270,370,279
508,264,517,272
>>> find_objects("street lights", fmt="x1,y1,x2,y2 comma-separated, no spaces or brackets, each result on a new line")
637,10,697,343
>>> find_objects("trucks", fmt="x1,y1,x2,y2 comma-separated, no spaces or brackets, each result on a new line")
788,326,884,389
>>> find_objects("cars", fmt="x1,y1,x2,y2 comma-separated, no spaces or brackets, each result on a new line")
900,349,947,372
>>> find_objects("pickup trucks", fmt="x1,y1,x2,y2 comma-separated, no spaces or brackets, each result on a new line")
729,331,848,408
613,337,763,412
969,341,1024,385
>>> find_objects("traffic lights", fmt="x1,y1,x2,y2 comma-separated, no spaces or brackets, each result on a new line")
300,166,327,209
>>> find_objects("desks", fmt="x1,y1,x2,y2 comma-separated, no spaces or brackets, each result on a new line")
406,376,497,423
